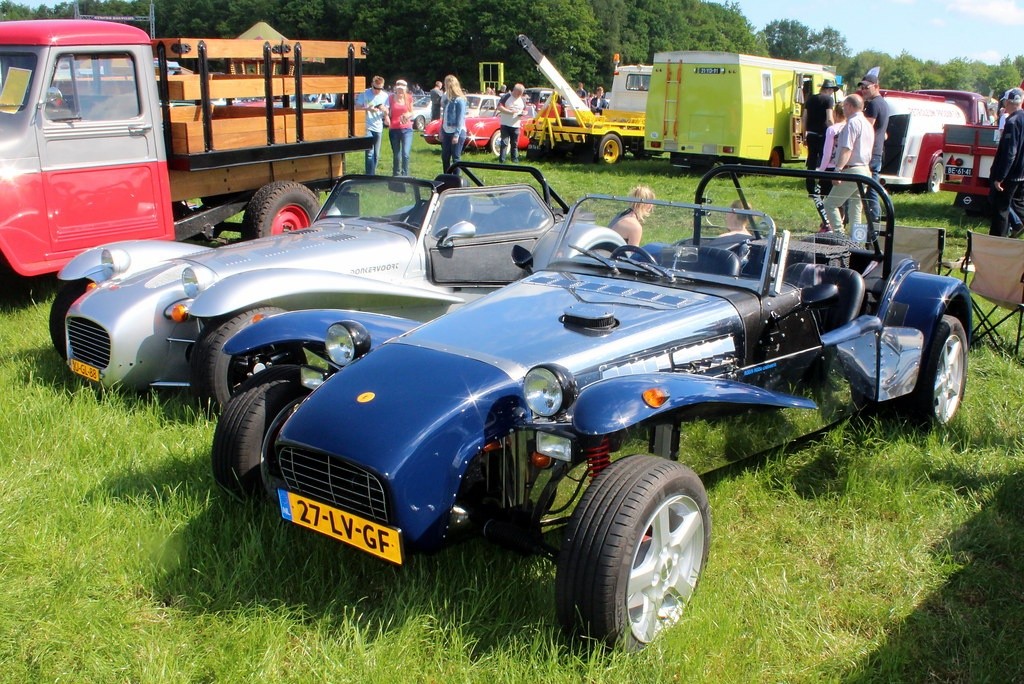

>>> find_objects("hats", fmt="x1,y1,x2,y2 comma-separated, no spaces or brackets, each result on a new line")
857,74,877,86
818,79,839,92
1000,87,1024,102
396,80,407,90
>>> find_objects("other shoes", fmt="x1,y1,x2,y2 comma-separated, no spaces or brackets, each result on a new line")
819,223,833,233
1009,224,1024,238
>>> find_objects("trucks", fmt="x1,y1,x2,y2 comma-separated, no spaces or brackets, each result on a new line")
942,78,1024,211
875,88,999,186
0,18,375,314
643,49,850,170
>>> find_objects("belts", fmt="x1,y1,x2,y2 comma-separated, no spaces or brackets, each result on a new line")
843,165,855,169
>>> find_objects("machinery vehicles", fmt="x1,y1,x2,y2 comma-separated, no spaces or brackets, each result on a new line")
516,32,653,163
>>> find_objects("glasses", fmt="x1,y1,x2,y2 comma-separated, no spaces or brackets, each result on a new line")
372,86,383,90
862,84,875,90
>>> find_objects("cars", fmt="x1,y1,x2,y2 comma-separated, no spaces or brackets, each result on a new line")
208,163,973,663
413,87,601,156
159,92,338,111
49,162,571,419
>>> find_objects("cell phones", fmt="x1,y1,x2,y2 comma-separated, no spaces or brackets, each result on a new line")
375,104,382,108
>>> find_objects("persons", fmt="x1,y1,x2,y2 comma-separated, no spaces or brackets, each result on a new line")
497,83,525,164
803,74,890,239
357,76,390,176
431,81,443,121
441,75,467,177
485,85,511,97
719,201,753,236
413,84,425,95
989,89,1024,238
610,187,655,255
385,80,414,176
576,81,608,114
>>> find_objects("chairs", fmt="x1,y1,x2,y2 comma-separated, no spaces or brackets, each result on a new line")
865,213,971,277
963,229,1023,363
780,262,866,333
405,173,470,239
653,243,741,277
477,183,541,236
